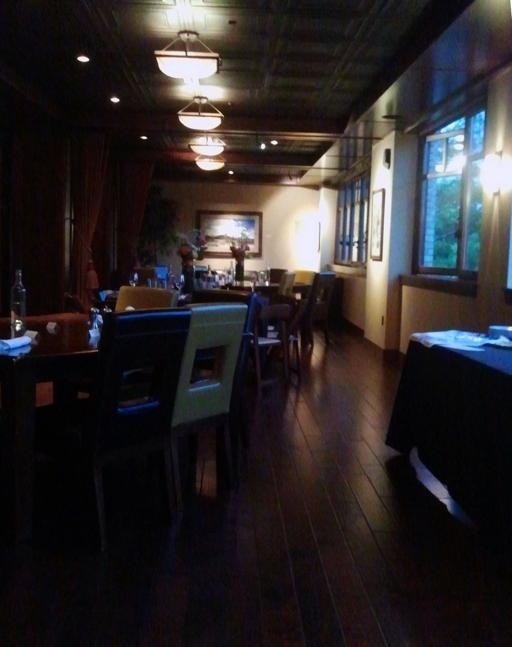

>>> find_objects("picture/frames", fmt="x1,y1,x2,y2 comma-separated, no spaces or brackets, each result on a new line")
196,209,263,258
368,189,387,262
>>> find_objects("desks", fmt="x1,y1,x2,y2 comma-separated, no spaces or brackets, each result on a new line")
385,329,512,577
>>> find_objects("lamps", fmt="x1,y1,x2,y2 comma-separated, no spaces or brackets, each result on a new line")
177,97,226,130
189,134,226,154
196,156,228,171
154,30,221,79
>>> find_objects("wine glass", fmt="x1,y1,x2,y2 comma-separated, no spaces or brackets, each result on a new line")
128,271,139,287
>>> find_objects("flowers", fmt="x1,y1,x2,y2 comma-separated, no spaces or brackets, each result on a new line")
229,243,251,266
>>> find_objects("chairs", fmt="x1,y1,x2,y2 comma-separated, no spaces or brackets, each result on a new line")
1,268,343,597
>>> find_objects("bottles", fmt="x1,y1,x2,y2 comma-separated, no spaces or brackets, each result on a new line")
10,269,26,324
226,260,234,285
206,264,213,288
